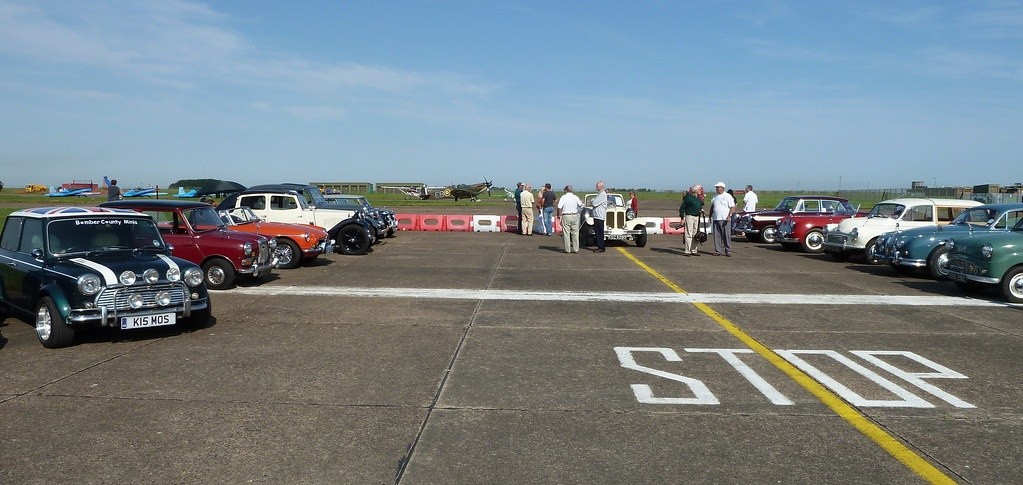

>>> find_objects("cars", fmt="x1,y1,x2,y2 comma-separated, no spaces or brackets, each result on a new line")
99,200,279,290
578,193,647,247
734,196,870,244
214,182,398,253
154,206,335,269
872,202,1023,304
0,206,211,349
820,197,988,265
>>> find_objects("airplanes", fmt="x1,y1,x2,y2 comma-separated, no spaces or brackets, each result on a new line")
42,185,101,198
173,187,224,198
381,184,448,200
123,186,168,197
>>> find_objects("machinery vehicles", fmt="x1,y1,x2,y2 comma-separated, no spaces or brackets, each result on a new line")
25,184,45,192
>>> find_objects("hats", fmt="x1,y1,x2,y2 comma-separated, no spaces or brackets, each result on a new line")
715,182,725,188
517,182,524,186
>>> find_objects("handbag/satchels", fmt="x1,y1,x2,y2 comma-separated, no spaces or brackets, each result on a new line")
694,232,707,242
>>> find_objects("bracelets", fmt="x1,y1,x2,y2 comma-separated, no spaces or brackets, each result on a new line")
730,214,732,216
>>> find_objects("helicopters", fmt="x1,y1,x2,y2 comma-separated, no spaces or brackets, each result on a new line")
451,177,492,202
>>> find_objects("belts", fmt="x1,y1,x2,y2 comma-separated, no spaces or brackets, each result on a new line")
562,212,579,215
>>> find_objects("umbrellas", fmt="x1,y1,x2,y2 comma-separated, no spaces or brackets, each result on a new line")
193,179,247,201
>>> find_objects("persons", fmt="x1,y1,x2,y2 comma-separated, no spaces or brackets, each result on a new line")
626,191,638,218
679,185,704,256
200,195,213,204
743,185,758,212
557,185,582,254
515,182,535,236
103,180,122,201
708,182,736,257
727,189,737,206
590,181,607,253
537,183,557,236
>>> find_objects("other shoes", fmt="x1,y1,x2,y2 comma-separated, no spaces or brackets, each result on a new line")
726,252,731,257
684,253,690,256
571,251,578,254
691,252,700,256
713,252,721,255
594,248,605,252
